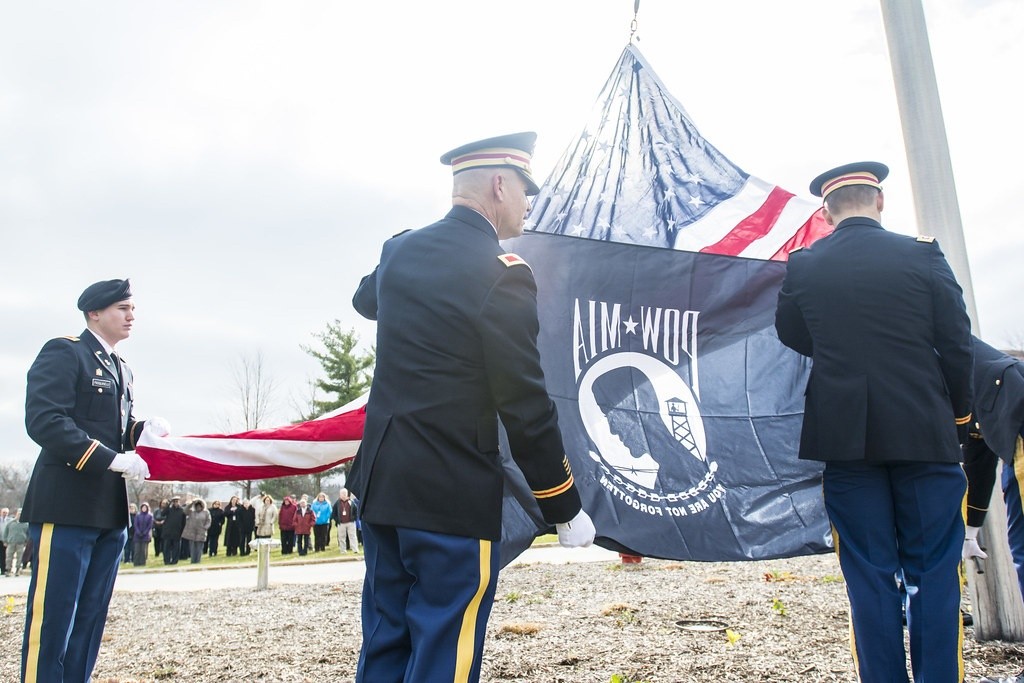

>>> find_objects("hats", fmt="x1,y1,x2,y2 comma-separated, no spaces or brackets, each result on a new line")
440,134,540,196
172,496,181,501
78,279,130,312
809,161,888,203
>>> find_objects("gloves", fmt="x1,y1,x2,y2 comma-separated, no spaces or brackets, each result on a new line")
143,418,168,438
554,506,596,548
961,539,988,563
108,451,150,483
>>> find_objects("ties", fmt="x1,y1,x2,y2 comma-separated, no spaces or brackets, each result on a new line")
112,353,119,373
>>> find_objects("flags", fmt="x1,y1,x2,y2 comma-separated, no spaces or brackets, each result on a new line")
116,58,847,486
433,220,871,565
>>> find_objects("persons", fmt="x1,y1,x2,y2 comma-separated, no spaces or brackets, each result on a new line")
0,488,365,577
344,131,596,683
18,278,170,683
951,334,1024,603
775,161,972,683
